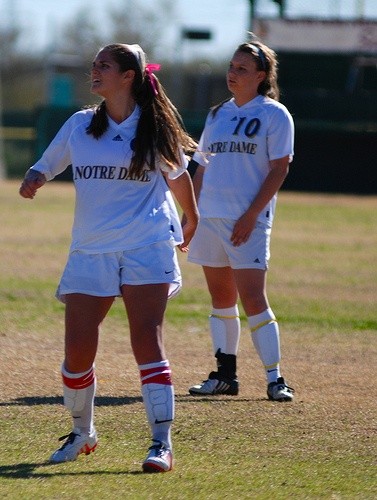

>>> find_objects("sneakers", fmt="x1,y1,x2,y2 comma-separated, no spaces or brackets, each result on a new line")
142,439,173,472
50,424,98,463
266,377,296,401
188,370,239,396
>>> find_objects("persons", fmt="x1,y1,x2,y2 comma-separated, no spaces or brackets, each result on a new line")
19,43,200,473
181,41,295,401
